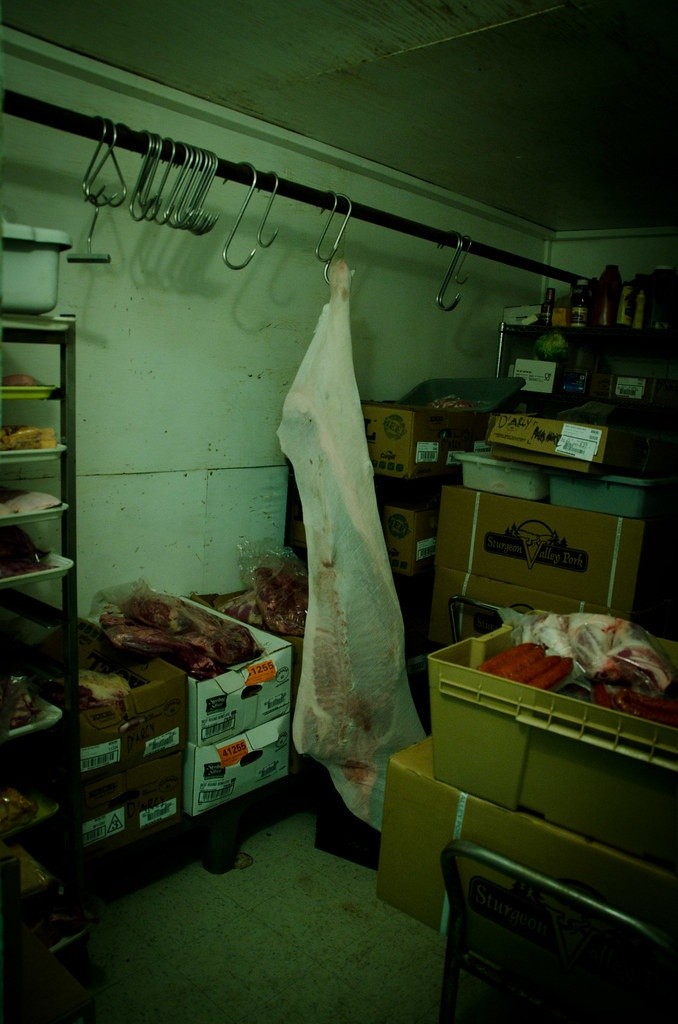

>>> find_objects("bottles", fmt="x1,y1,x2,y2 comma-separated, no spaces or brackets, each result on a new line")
539,287,556,326
632,288,645,329
615,282,637,329
569,278,592,326
591,265,623,327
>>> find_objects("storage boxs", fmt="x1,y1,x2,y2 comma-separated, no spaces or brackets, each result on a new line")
0,220,71,315
36,374,678,982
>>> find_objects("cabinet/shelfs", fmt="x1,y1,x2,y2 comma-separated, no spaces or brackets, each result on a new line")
0,313,92,941
495,323,678,415
